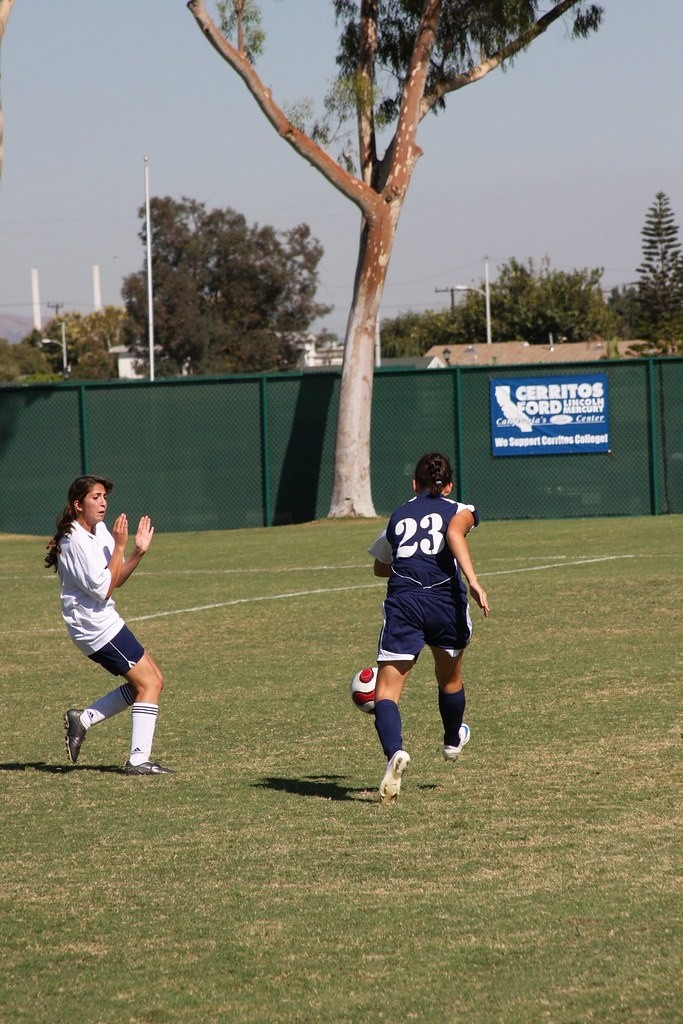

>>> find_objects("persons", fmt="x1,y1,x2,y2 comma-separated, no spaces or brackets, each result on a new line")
44,474,173,774
367,452,490,805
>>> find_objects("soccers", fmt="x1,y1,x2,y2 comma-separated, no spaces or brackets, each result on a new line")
350,667,380,713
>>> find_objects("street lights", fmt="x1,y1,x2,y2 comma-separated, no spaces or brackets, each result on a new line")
41,322,69,380
454,261,492,343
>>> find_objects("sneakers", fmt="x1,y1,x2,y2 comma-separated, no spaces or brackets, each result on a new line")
123,761,176,776
441,722,470,762
63,708,87,764
378,750,411,806
